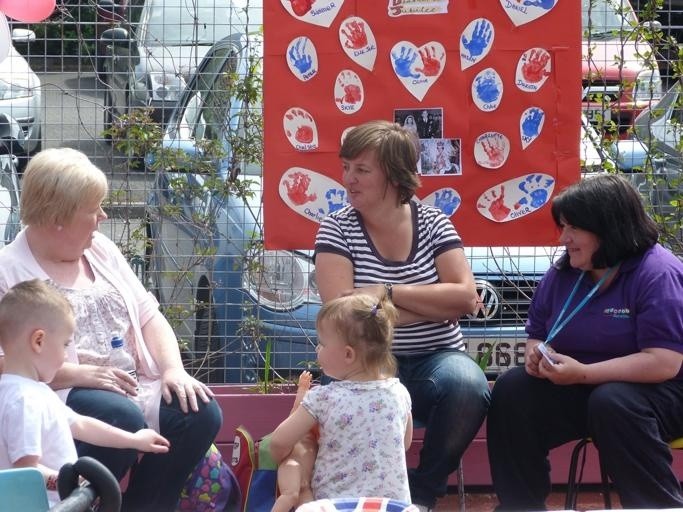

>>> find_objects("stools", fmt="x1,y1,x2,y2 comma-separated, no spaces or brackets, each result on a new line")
565,433,683,511
411,415,465,512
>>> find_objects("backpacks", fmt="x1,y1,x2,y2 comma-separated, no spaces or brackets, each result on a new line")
183,443,242,512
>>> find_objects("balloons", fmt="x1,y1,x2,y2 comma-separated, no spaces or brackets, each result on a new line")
0,1,58,22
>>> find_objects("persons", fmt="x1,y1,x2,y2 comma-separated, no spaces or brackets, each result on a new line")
1,144,222,512
269,369,326,512
1,279,173,511
482,172,682,509
417,110,437,138
306,119,493,511
402,115,417,133
270,292,417,505
424,140,448,176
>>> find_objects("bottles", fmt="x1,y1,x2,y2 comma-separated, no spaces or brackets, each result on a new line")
105,331,139,407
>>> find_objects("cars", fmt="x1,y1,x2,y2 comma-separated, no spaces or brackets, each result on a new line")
615,81,683,255
582,0,664,139
0,8,44,156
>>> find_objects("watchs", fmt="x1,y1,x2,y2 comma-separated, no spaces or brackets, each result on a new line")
382,280,394,300
